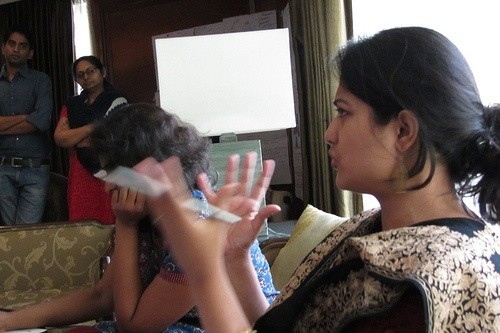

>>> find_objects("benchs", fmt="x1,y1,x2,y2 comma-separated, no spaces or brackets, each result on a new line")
0,219,116,323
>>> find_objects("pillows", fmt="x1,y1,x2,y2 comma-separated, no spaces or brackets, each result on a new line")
270,204,349,291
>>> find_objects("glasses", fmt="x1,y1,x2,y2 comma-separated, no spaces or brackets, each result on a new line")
74,66,98,79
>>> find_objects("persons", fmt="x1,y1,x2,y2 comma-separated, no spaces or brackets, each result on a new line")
53,55,130,225
0,101,281,333
0,24,58,225
130,27,500,333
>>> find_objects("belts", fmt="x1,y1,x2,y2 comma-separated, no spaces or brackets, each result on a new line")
0,155,52,168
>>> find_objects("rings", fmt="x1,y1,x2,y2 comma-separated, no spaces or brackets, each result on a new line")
152,211,169,223
